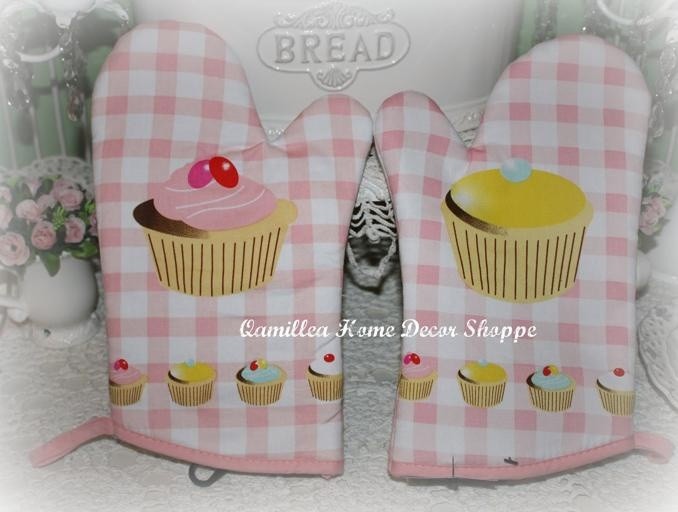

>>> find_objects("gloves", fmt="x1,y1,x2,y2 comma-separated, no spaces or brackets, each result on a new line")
27,19,373,488
374,32,672,483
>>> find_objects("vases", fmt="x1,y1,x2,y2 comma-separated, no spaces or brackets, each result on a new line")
23,254,99,350
635,248,654,299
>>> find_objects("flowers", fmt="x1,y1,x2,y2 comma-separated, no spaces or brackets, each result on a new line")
638,174,671,254
0,171,99,277
